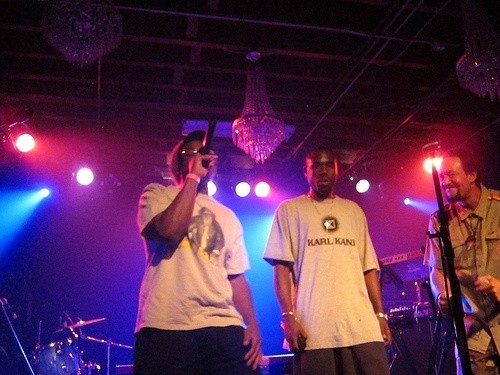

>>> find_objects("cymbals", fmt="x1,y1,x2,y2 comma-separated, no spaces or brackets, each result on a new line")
53,316,107,335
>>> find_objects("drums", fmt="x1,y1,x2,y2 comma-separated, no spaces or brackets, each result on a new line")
30,337,84,375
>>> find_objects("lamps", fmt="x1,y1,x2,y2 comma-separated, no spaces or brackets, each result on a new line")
456,33,500,101
232,52,285,165
421,141,444,174
0,118,35,152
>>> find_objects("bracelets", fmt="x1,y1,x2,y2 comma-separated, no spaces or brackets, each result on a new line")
186,173,200,184
282,312,294,316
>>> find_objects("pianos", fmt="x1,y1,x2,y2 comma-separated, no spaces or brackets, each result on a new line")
376,244,427,303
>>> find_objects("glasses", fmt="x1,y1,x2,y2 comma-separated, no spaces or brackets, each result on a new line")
180,148,200,159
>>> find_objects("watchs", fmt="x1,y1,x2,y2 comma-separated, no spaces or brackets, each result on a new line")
376,313,388,320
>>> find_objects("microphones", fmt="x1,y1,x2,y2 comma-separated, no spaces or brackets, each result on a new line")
199,119,217,168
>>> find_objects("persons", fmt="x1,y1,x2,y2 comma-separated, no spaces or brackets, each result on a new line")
423,148,500,375
263,148,393,375
134,131,262,375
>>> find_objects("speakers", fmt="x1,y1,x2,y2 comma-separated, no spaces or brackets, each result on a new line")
377,250,459,375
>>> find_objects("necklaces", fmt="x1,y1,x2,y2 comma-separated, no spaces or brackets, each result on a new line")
309,194,335,232
